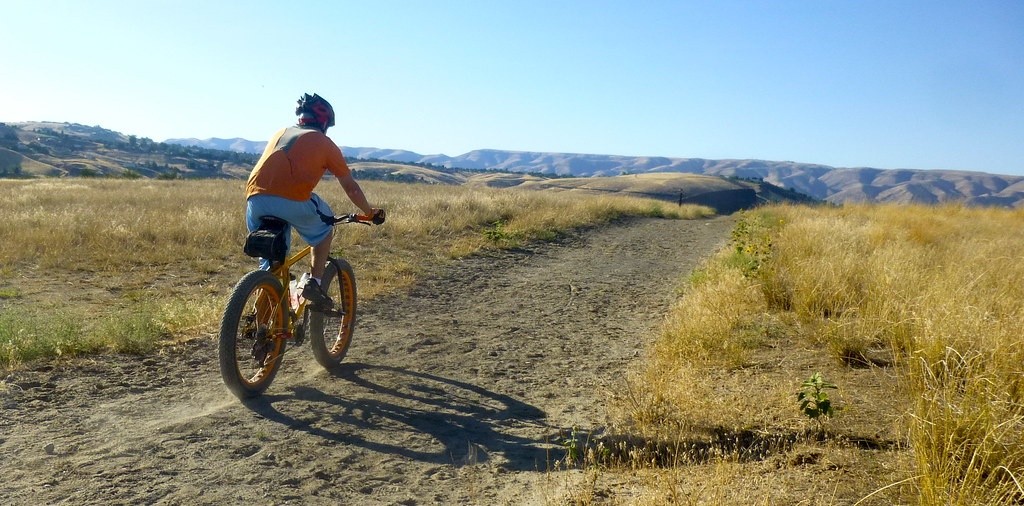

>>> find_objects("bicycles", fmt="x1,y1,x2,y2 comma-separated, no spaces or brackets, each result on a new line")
217,211,374,400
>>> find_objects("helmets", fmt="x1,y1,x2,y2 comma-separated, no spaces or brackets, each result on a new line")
295,93,335,128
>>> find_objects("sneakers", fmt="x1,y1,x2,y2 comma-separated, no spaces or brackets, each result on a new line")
301,278,334,310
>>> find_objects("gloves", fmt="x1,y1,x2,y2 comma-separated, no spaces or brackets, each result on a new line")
368,207,386,225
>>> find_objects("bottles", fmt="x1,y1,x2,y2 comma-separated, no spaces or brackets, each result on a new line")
289,273,298,309
297,272,310,296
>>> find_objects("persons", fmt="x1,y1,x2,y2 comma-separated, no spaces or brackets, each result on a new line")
245,92,386,317
